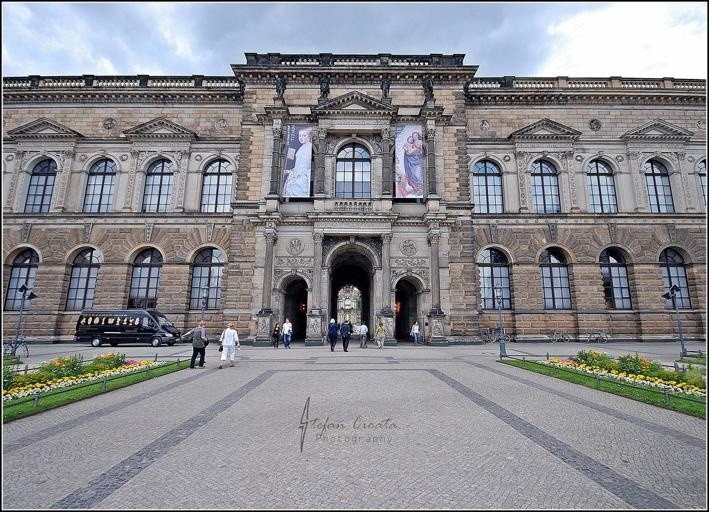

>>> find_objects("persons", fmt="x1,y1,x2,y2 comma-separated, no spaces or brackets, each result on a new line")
283,127,311,197
412,320,420,344
404,132,423,195
340,319,352,352
218,321,240,369
273,321,281,348
403,136,420,156
376,321,388,349
182,320,209,369
328,318,338,351
281,318,293,350
356,321,369,348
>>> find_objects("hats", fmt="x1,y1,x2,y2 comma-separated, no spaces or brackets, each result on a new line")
197,319,206,326
225,321,235,328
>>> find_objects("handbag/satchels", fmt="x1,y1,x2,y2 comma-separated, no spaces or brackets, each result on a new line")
219,344,224,352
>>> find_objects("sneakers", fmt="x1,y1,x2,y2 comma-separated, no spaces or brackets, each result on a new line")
330,344,385,353
188,363,236,369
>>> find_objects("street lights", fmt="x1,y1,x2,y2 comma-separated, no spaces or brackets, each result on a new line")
495,281,507,357
201,282,210,321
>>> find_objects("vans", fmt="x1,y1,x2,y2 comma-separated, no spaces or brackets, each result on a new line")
74,308,182,347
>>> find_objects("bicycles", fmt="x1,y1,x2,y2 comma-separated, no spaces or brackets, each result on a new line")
587,328,608,344
362,335,366,348
550,329,570,343
3,337,29,358
481,326,511,343
